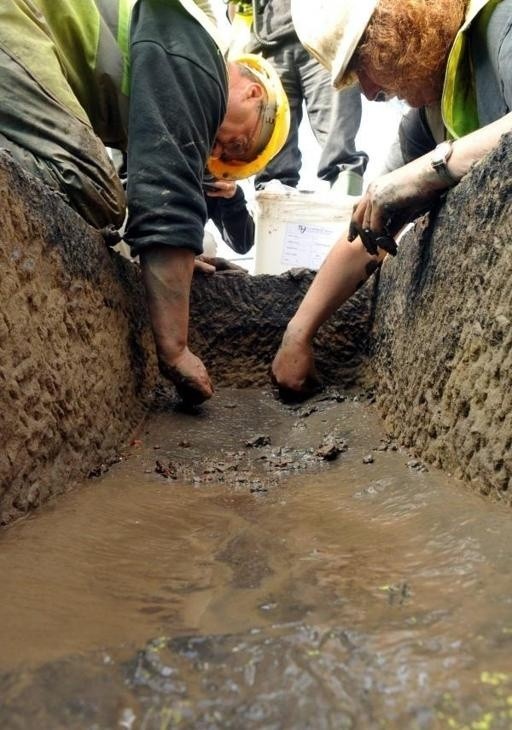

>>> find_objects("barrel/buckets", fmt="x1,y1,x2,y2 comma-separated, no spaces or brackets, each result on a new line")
253,190,362,276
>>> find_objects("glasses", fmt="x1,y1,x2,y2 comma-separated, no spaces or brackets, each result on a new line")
223,97,264,151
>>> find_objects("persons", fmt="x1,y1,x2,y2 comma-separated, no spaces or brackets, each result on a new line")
262,1,511,399
0,1,289,404
194,0,366,200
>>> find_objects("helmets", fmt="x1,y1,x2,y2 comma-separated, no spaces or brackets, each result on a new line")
206,52,292,181
290,1,379,85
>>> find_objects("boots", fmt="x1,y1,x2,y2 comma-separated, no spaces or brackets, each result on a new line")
328,170,364,196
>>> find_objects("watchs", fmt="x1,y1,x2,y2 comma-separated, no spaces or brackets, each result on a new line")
431,138,458,186
